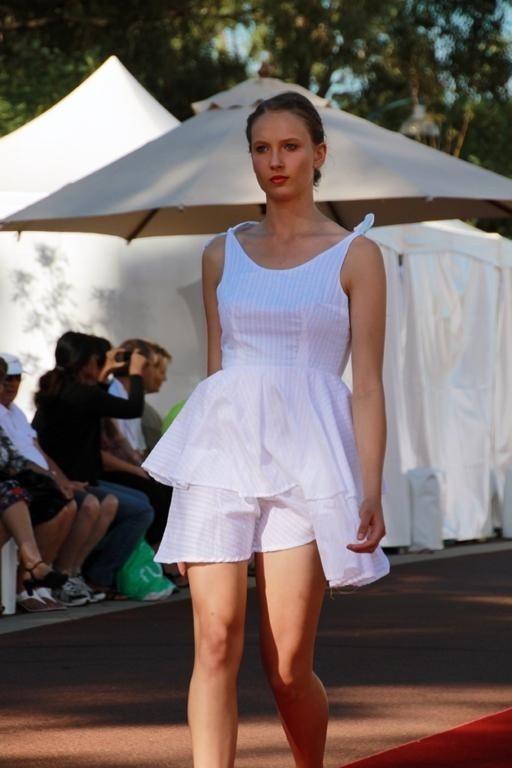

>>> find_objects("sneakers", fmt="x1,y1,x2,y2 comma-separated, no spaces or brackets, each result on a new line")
16,575,106,612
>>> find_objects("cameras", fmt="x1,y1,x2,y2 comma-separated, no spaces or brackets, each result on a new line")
117,349,134,362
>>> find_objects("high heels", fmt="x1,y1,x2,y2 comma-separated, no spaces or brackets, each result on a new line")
22,560,68,596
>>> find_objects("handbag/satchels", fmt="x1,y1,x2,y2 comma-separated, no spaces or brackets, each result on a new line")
115,532,179,602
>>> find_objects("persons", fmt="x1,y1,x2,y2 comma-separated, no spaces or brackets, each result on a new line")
30,333,187,599
1,354,118,606
2,359,78,610
141,93,388,768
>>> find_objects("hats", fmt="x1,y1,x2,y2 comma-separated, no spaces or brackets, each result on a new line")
0,352,30,376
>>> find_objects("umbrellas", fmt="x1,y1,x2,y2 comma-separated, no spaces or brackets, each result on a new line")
0,75,512,245
